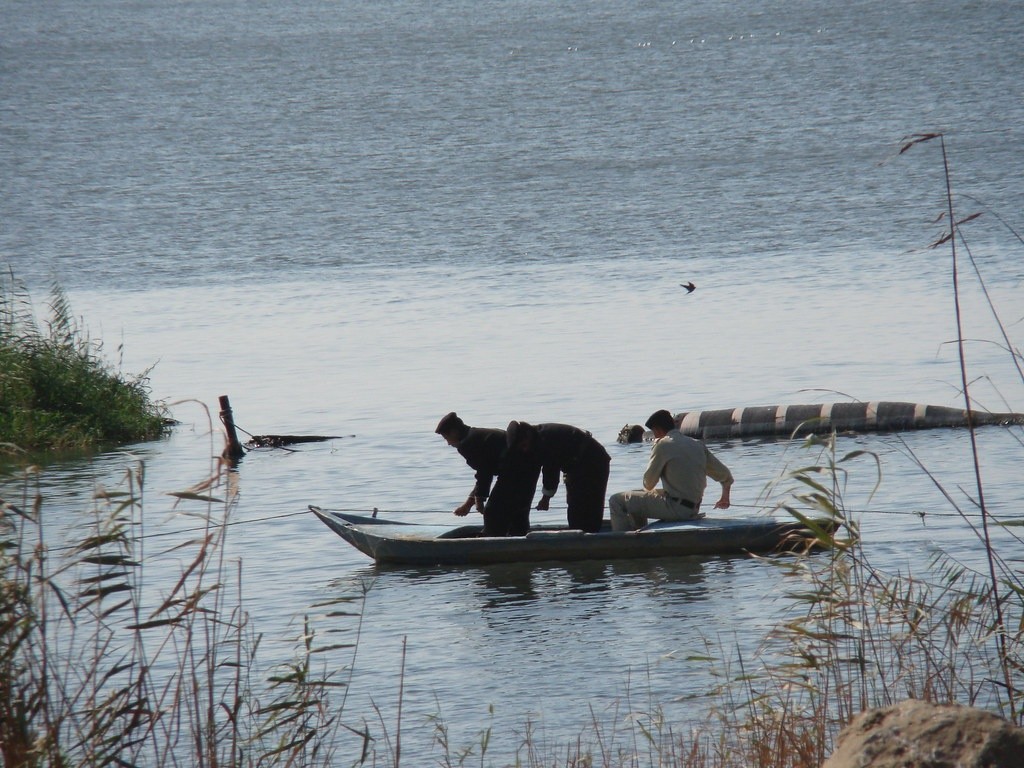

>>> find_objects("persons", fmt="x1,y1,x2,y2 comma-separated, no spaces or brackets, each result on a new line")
610,410,734,529
434,412,542,536
507,420,611,533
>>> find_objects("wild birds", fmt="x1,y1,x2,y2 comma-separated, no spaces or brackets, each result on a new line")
678,281,698,295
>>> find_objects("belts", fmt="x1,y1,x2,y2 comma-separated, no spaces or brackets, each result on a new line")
662,495,700,509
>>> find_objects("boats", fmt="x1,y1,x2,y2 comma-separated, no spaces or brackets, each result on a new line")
309,504,843,565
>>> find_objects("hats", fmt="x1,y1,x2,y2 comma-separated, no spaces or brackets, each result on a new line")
506,420,518,449
645,410,670,429
435,411,456,434
